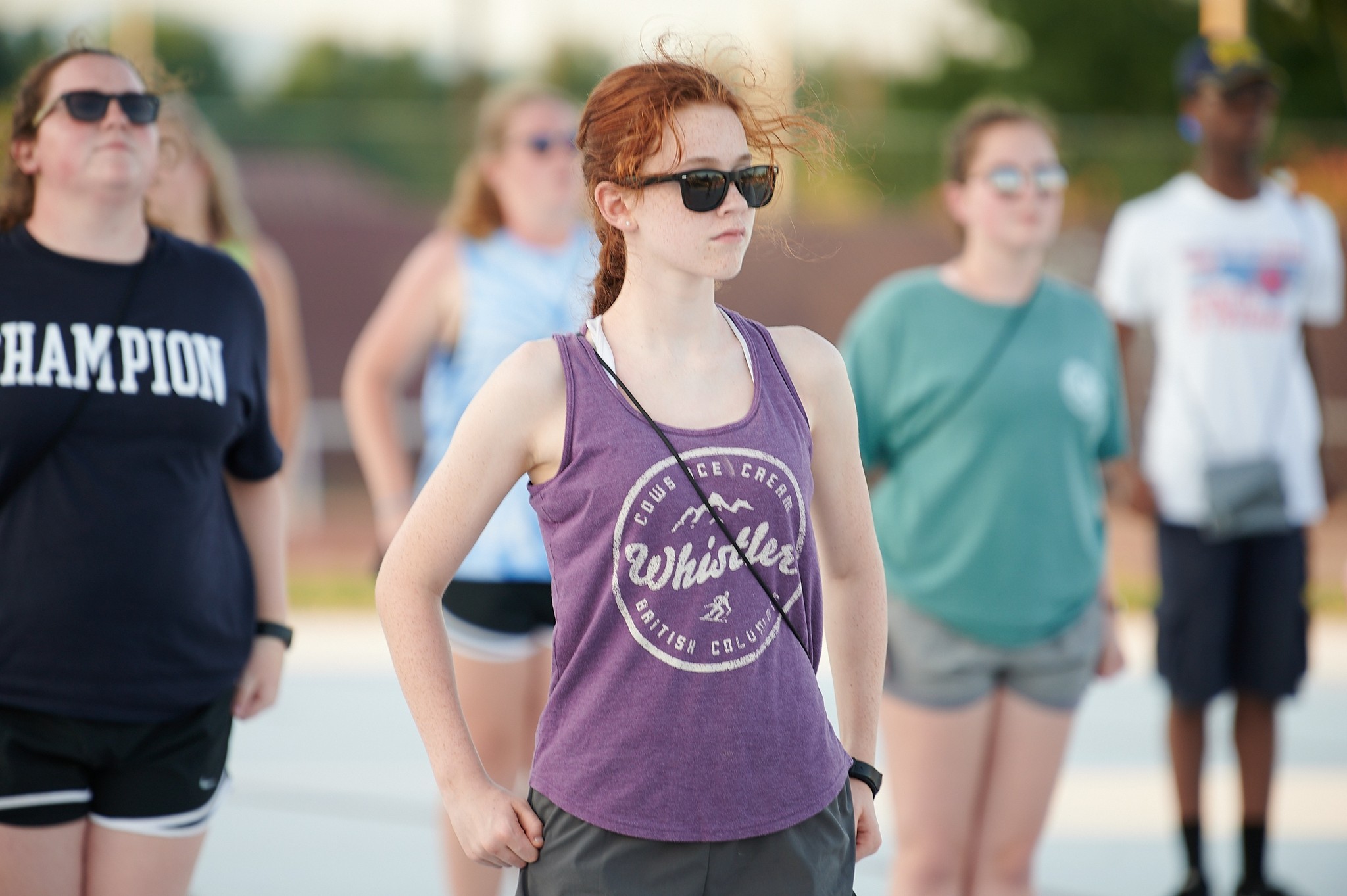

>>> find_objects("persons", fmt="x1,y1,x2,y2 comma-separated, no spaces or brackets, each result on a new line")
835,96,1136,896
371,31,891,896
1095,30,1347,896
0,48,311,896
336,75,602,896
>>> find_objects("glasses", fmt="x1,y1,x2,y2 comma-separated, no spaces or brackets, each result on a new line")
525,132,582,161
985,164,1069,201
35,87,161,131
624,165,780,212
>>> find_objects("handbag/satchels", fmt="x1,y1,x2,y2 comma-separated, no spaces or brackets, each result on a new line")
1205,460,1291,541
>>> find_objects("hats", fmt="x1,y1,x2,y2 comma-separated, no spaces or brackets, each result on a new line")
1173,34,1283,101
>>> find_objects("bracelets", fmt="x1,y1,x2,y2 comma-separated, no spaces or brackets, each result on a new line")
253,619,293,648
847,755,882,800
